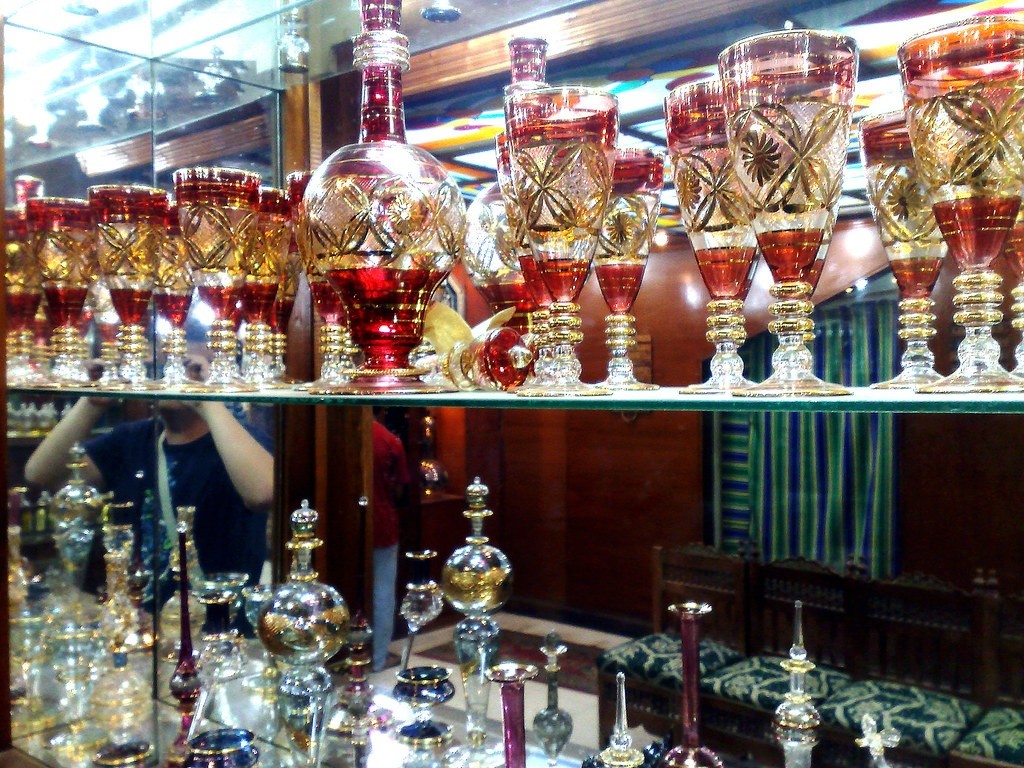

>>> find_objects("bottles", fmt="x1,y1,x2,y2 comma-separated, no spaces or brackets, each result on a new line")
5,439,899,768
468,38,545,334
304,0,463,376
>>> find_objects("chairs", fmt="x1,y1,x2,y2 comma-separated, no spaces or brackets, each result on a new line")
593,541,1024,768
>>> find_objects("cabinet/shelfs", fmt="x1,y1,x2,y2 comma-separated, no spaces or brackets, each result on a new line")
0,378,1022,766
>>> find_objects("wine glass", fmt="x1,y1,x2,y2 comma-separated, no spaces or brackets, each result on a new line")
1,0,1024,392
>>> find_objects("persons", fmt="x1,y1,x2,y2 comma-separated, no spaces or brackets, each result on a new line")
24,324,274,639
373,406,411,549
